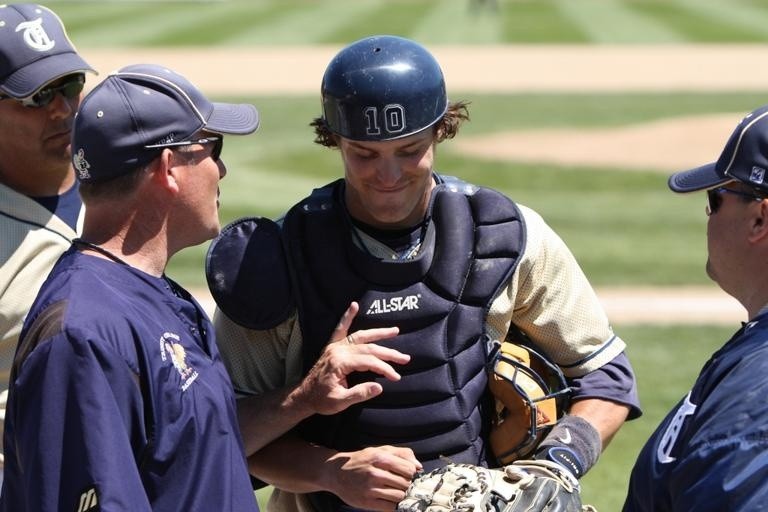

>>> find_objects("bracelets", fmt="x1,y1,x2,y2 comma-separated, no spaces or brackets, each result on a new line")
537,411,601,476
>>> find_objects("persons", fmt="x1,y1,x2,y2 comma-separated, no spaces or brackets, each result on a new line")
202,36,638,512
0,1,109,444
619,99,767,510
0,63,409,512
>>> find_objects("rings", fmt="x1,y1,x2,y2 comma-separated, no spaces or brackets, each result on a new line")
346,334,355,346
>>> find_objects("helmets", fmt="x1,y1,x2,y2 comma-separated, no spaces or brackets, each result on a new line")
320,36,449,142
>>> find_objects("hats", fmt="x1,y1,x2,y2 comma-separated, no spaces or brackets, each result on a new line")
70,63,260,185
667,105,767,193
0,3,99,101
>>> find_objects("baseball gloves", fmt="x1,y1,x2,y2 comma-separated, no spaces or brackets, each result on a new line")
397,463,601,512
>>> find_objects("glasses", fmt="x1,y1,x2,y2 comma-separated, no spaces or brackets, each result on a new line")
143,134,223,162
0,73,85,109
705,186,764,216
